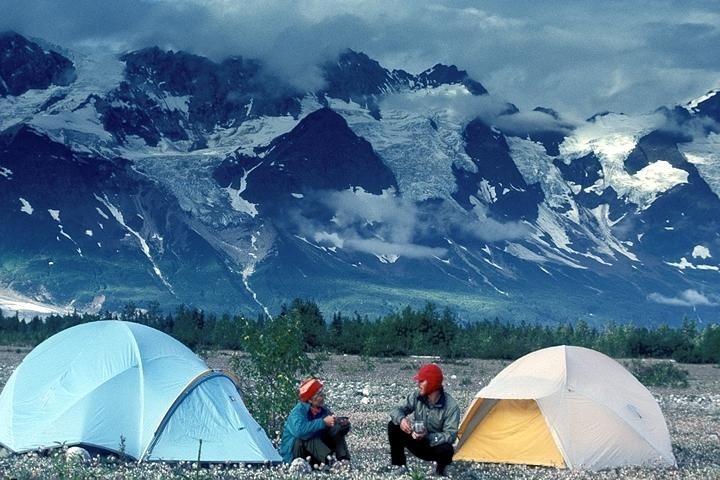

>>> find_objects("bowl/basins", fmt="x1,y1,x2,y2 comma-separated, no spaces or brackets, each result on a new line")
336,416,349,424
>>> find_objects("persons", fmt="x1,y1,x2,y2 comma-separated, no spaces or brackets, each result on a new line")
280,375,365,462
386,362,463,477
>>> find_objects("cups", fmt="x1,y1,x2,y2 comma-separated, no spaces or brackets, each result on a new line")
410,420,425,434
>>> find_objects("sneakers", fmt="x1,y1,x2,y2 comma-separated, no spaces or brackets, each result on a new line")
324,455,350,472
392,464,408,475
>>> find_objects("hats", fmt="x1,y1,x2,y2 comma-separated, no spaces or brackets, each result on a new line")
298,378,323,402
412,365,442,395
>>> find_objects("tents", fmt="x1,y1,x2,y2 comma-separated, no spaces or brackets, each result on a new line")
450,343,681,471
1,317,287,467
